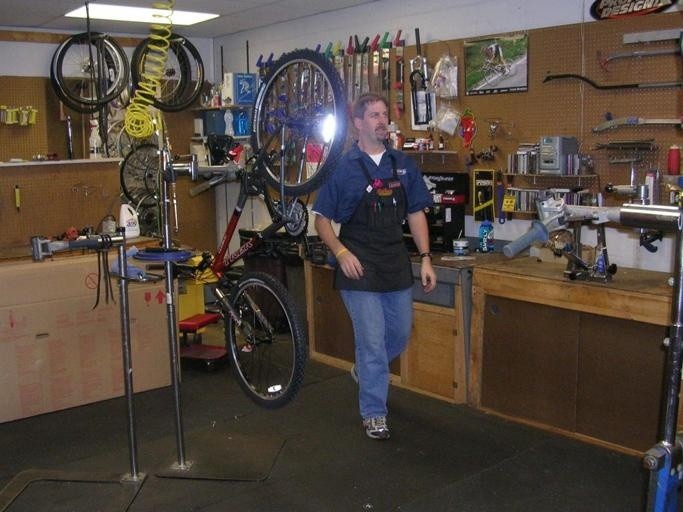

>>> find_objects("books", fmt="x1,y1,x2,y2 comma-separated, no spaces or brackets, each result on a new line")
499,142,604,213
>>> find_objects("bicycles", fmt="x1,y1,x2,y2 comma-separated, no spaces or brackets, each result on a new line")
159,48,351,409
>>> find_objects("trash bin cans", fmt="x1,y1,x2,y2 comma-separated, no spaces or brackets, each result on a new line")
238,228,288,319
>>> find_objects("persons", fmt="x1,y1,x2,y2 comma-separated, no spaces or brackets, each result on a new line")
479,43,509,76
475,189,488,219
308,91,438,443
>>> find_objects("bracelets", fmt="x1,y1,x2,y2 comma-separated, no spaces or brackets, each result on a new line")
419,252,435,261
334,247,349,258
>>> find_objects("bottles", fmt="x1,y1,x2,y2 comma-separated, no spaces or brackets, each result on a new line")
87,119,104,160
436,133,443,149
643,167,659,205
667,143,680,176
477,218,495,253
428,133,433,149
223,109,235,136
236,106,248,135
418,140,424,151
395,130,402,150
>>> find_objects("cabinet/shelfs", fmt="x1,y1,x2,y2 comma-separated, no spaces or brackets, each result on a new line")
0,235,181,424
470,256,681,462
187,103,293,171
303,249,537,407
499,172,597,217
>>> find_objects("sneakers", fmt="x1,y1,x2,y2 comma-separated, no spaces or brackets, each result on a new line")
363,415,390,440
351,364,360,384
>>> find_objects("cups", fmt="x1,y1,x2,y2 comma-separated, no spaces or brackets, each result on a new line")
450,238,469,257
